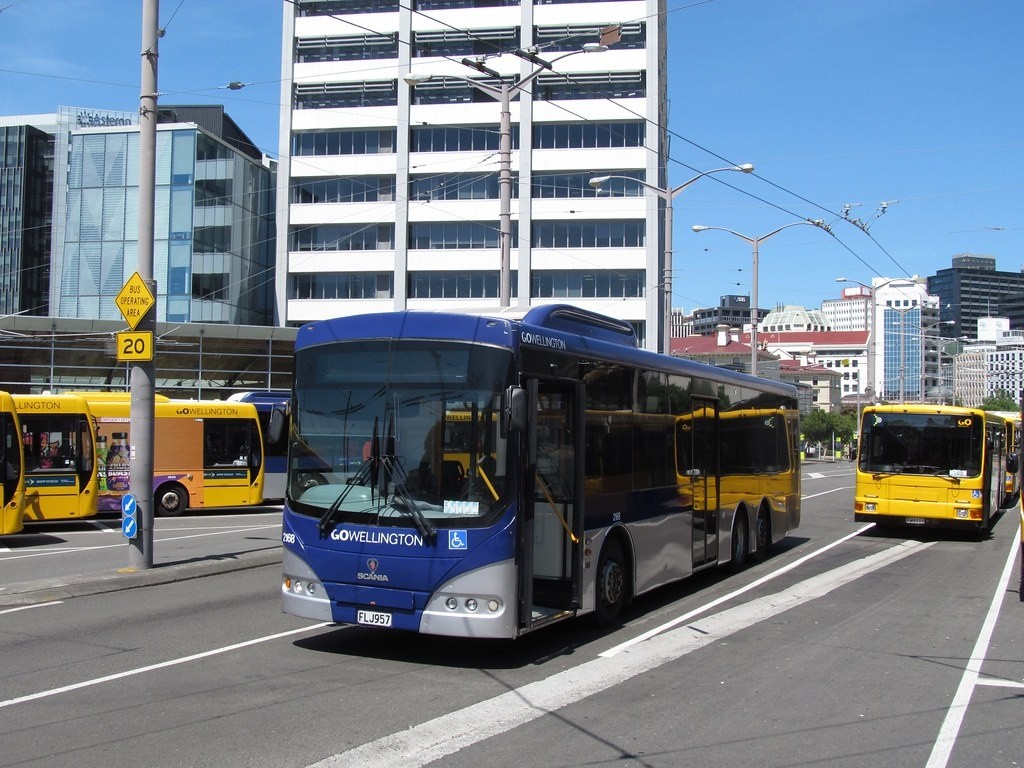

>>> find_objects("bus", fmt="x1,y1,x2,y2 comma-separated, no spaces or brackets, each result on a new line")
533,408,677,494
673,410,802,513
855,403,1009,533
279,305,802,642
442,410,499,478
225,388,390,499
1007,388,1024,601
60,392,267,517
11,394,100,522
0,391,25,535
1005,418,1022,503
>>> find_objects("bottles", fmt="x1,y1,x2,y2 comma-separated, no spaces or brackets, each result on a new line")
95,436,109,491
106,432,130,491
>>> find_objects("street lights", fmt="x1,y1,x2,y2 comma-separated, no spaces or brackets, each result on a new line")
692,219,823,377
588,164,754,357
892,321,956,404
930,349,980,406
870,301,938,405
835,278,917,407
912,336,967,406
403,43,610,308
